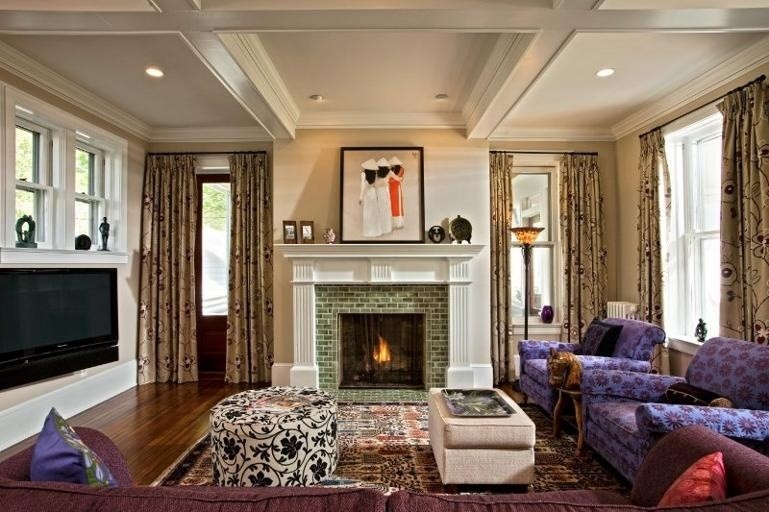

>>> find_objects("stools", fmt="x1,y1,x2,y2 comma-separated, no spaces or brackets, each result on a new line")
209,384,342,490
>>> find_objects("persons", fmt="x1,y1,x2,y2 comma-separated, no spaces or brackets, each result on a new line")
370,156,405,236
357,157,383,240
383,155,406,231
97,217,110,251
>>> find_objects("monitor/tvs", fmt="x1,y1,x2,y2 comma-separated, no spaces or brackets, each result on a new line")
0,267,119,392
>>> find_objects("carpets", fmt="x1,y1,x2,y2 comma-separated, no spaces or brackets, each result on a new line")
150,402,631,495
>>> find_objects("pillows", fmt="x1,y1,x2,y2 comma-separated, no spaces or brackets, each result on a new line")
667,383,734,412
30,407,119,486
657,452,727,508
580,317,623,357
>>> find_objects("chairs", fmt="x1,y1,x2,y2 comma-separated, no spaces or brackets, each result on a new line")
564,336,769,487
517,317,665,438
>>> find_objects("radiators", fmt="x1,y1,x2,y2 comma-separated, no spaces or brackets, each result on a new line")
607,301,642,320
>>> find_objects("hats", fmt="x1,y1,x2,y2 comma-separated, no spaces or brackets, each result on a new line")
360,158,379,170
376,157,390,167
387,156,403,165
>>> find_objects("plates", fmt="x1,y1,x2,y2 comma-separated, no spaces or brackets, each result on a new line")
429,225,445,244
449,218,472,240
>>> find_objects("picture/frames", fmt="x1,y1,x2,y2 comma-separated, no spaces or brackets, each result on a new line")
338,146,426,246
300,221,315,244
282,220,297,244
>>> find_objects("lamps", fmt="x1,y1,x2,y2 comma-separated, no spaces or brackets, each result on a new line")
511,227,547,340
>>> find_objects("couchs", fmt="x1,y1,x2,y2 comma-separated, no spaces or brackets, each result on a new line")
0,423,768,510
